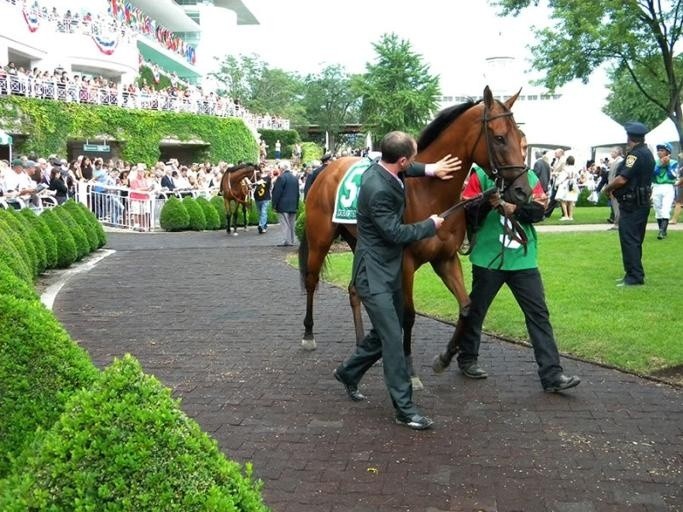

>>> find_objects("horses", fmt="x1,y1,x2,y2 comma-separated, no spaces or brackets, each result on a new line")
219,162,263,236
298,85,534,391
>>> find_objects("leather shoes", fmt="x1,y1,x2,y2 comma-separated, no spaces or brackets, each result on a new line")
460,362,488,379
333,368,367,402
544,373,580,394
395,415,434,430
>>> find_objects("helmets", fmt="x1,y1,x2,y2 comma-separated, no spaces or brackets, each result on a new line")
656,143,673,153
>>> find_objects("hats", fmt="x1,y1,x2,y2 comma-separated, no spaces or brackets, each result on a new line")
23,160,40,168
625,122,650,137
14,159,23,166
321,154,331,161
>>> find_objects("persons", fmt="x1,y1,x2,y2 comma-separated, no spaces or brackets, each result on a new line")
332,130,463,430
456,127,582,394
1,0,371,235
530,139,683,240
600,121,656,286
271,161,300,246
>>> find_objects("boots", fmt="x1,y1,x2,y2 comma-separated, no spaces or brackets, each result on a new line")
657,218,669,240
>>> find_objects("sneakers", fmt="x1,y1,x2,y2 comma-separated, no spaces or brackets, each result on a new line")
617,278,644,286
560,216,573,221
277,240,296,246
257,225,267,234
